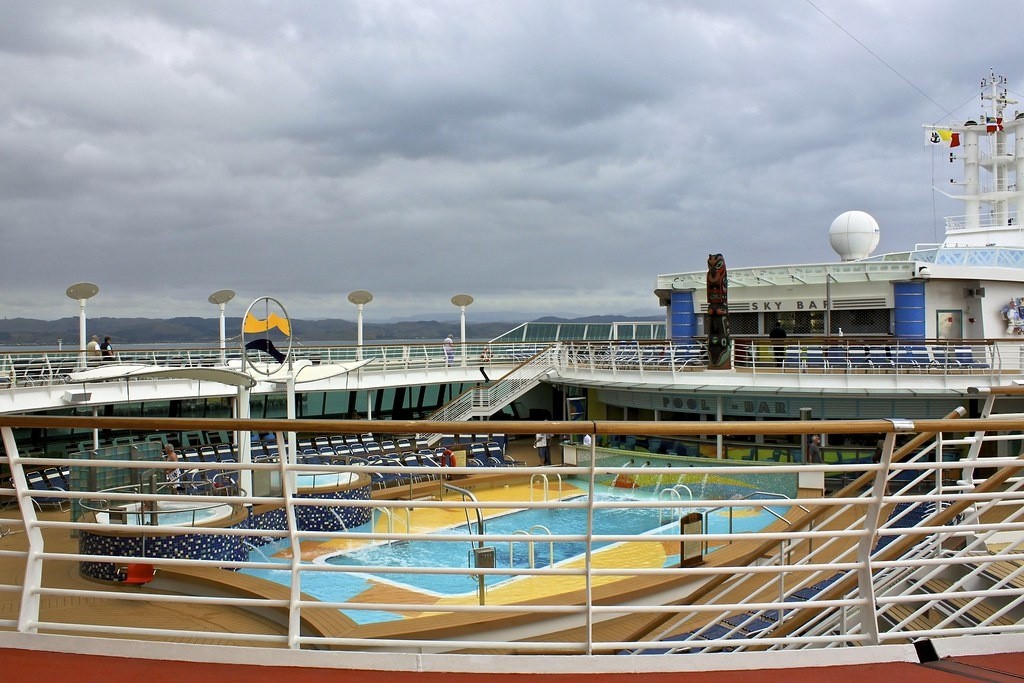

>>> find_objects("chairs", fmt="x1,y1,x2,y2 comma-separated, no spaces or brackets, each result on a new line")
507,340,706,373
1,350,324,389
7,410,527,511
785,346,990,374
606,435,801,462
121,564,161,584
616,499,966,655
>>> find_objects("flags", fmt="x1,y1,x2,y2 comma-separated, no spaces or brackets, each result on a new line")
925,130,960,148
986,117,1003,133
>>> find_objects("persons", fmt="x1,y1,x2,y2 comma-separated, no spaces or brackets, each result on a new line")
138,501,161,511
534,419,554,467
583,434,592,446
100,337,115,361
164,443,180,494
481,346,494,362
872,440,891,496
87,334,103,367
769,322,786,367
443,334,455,367
808,434,824,463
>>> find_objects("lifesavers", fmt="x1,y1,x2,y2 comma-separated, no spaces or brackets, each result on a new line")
442,450,457,467
